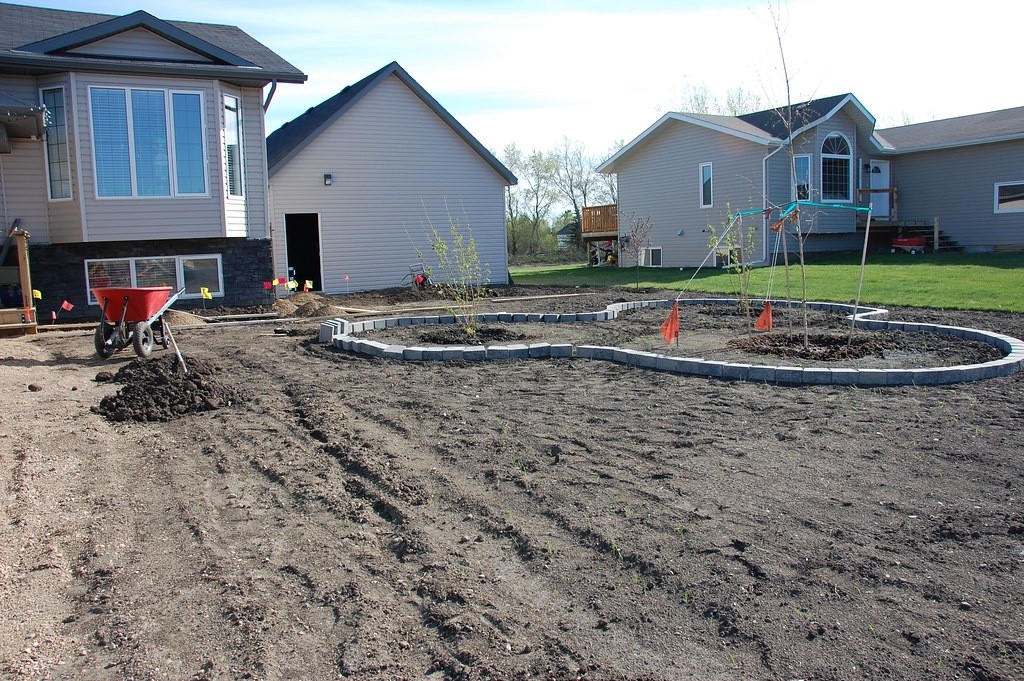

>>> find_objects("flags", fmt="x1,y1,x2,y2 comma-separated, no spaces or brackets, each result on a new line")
263,277,313,292
33,290,42,299
51,311,57,320
201,287,212,299
756,302,772,331
62,300,74,311
659,303,678,343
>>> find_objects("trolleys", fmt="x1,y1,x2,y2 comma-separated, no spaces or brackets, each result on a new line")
91,284,186,361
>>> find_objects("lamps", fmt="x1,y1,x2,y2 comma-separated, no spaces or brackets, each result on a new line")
324,175,332,185
865,163,870,173
